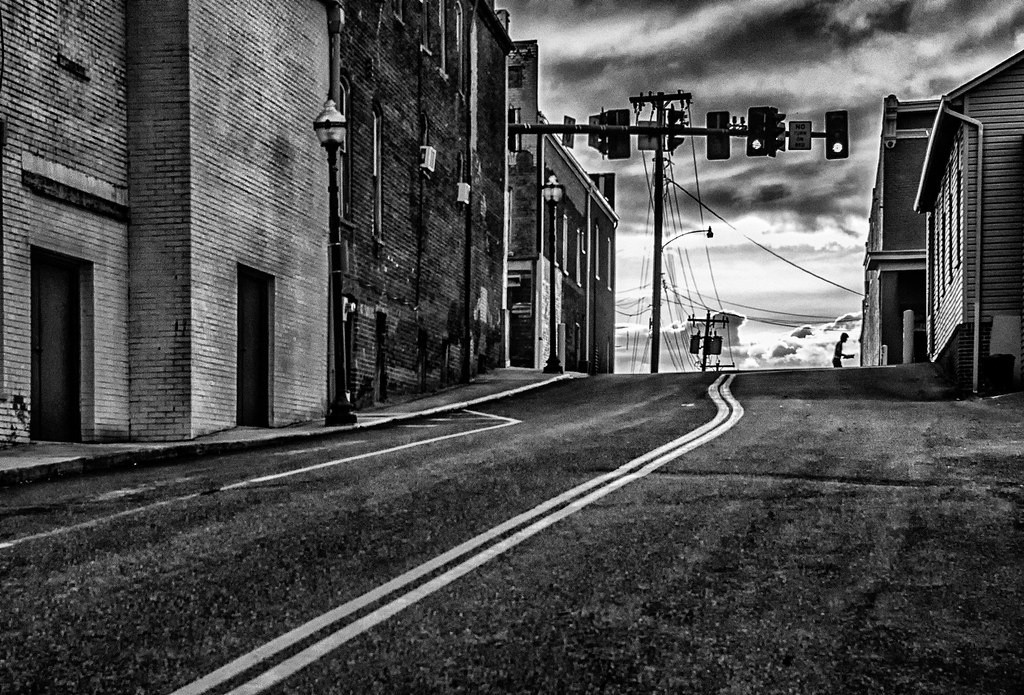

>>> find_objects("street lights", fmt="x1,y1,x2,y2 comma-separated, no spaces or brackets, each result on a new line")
629,89,713,374
542,175,564,374
313,99,358,426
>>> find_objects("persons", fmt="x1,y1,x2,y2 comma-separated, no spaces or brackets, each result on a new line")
833,333,849,367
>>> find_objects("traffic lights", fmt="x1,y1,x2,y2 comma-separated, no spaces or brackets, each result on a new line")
747,107,786,157
825,111,849,159
